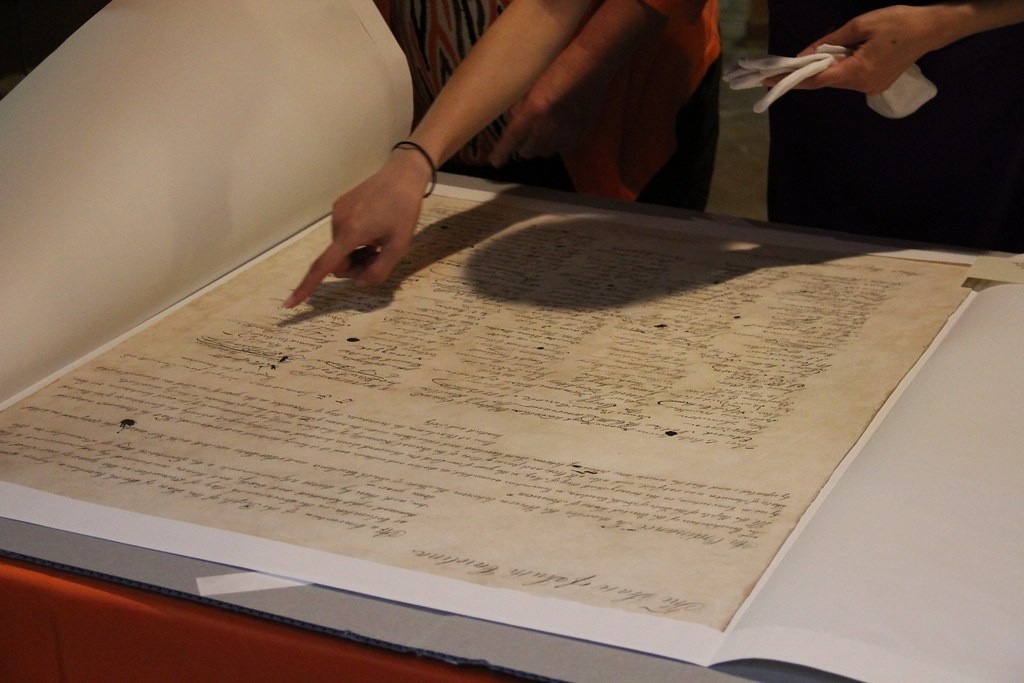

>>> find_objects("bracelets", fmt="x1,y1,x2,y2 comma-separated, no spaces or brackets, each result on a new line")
391,141,436,199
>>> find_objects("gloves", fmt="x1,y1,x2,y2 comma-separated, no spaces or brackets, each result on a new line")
723,43,938,119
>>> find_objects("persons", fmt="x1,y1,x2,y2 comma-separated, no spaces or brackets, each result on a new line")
283,1,1023,310
